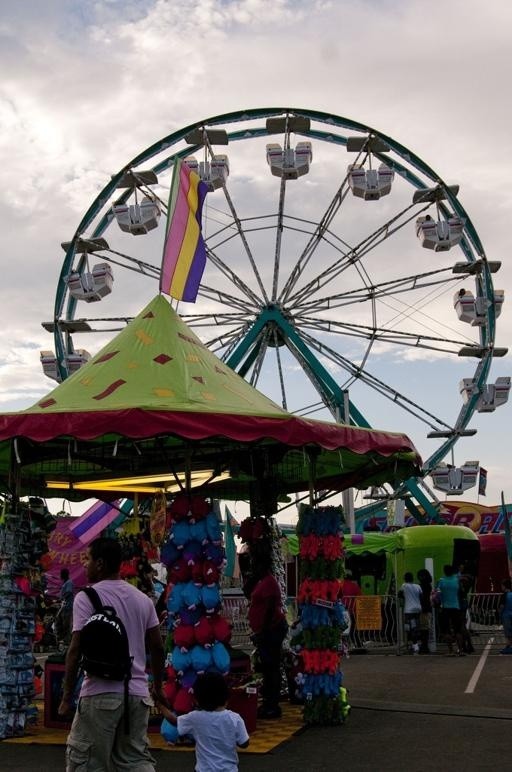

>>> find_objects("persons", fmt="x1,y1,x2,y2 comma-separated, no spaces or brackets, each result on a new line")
422,215,434,225
55,569,74,630
143,195,151,201
459,289,466,298
58,536,164,771
156,671,250,772
397,561,474,657
245,556,288,720
448,214,456,224
497,577,512,654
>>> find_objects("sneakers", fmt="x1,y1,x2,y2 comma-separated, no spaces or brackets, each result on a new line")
500,645,512,653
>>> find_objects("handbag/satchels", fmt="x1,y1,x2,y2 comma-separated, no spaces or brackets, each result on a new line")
397,590,405,607
431,588,441,608
78,606,133,681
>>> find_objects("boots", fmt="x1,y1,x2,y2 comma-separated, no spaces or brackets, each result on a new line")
455,633,466,656
446,634,455,656
